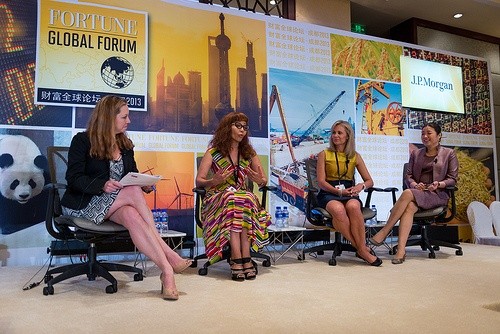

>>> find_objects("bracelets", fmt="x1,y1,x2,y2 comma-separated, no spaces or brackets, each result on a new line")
362,184,366,189
339,189,342,197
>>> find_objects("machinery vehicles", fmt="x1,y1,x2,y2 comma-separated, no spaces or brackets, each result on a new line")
270,85,309,210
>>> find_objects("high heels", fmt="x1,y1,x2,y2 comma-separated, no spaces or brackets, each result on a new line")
160,273,178,300
173,258,193,273
229,257,256,281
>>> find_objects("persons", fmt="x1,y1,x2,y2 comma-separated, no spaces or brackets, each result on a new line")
317,120,383,266
369,123,459,264
196,113,272,281
59,96,193,299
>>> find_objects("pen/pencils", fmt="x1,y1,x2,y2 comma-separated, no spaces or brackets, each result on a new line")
151,185,156,191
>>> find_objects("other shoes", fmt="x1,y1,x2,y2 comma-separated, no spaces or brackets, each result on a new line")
355,245,382,266
368,236,386,246
392,250,407,264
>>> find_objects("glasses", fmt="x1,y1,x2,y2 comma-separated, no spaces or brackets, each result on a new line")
231,121,250,131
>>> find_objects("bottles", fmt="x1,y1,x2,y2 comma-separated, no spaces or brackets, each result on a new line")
152,209,168,233
275,206,289,228
371,205,377,225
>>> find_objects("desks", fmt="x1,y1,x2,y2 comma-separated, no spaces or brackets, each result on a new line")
265,224,307,266
362,221,394,260
133,230,186,276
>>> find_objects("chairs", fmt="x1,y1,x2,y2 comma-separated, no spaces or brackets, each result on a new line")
466,200,500,246
297,158,377,267
42,146,143,295
385,163,465,256
192,157,277,273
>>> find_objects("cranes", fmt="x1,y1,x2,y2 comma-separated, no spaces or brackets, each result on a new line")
290,91,345,147
356,79,389,134
310,104,323,135
